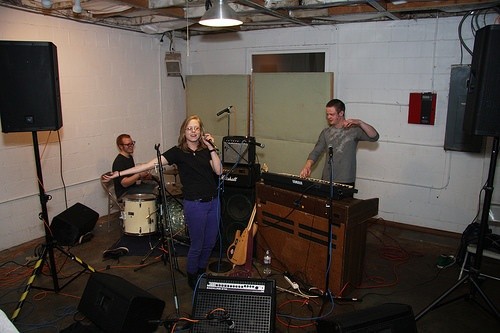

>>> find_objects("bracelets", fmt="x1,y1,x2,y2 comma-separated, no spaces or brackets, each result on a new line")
119,171,120,176
209,149,216,152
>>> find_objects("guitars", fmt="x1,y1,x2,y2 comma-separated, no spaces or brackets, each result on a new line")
227,203,257,265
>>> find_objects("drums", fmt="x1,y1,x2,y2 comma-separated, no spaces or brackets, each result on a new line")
163,197,192,241
123,193,159,236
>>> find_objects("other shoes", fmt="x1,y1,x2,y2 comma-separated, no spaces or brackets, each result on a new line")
437,254,456,269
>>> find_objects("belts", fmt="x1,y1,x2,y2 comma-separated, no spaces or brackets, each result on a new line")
185,196,218,202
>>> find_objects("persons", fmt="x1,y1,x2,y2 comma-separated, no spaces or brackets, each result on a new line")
301,99,379,187
112,133,159,200
103,114,223,289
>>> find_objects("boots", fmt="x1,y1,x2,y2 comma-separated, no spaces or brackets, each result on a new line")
187,272,198,290
198,267,206,275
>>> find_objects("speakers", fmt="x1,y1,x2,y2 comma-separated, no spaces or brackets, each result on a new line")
0,40,63,133
215,188,256,255
222,136,256,166
77,272,166,333
443,23,500,154
50,203,99,246
316,302,417,333
189,272,276,333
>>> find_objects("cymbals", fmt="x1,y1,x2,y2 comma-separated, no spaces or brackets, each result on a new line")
163,168,179,176
153,183,182,195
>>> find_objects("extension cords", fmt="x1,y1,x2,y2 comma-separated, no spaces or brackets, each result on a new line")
285,275,299,290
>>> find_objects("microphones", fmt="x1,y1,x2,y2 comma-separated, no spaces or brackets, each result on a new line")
247,141,265,148
328,144,333,157
204,135,217,149
224,310,235,329
216,106,233,117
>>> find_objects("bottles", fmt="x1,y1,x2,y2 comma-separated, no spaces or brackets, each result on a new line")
263,250,271,274
261,162,268,182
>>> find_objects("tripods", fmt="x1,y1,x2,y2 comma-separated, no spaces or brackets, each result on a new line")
415,137,500,333
10,131,97,325
134,176,193,277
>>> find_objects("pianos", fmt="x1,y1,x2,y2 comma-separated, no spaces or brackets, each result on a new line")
263,171,359,200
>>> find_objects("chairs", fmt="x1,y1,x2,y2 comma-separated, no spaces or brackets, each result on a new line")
100,171,126,232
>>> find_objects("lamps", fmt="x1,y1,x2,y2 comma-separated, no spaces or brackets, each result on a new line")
41,0,52,9
72,0,82,13
198,0,244,27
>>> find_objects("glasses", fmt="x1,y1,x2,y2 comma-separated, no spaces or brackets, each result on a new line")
185,127,201,131
120,141,135,147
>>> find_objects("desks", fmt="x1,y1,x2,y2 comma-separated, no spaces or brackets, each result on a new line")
258,200,366,294
